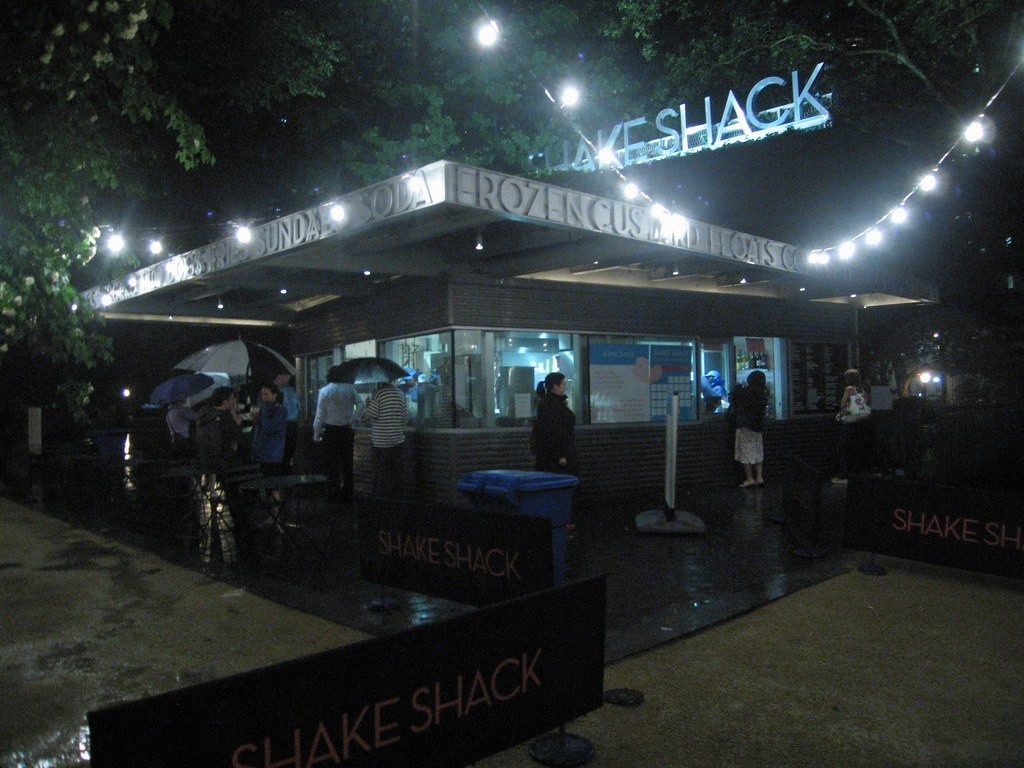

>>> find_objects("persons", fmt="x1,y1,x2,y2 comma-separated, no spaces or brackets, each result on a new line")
403,367,423,418
831,368,870,483
250,376,297,476
165,406,196,451
366,382,409,496
732,370,769,488
196,386,260,535
313,366,366,503
531,372,581,540
699,370,727,413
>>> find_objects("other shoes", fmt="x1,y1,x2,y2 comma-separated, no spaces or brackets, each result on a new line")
561,522,575,530
258,520,286,533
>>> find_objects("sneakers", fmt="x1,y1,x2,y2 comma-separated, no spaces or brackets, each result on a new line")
831,476,848,485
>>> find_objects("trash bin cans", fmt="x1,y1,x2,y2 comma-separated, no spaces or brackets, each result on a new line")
458,470,580,584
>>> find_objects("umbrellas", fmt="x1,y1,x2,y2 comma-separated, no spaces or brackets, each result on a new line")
148,374,216,405
324,358,410,398
172,339,300,419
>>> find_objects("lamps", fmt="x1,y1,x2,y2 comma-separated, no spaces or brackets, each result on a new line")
97,1,1023,266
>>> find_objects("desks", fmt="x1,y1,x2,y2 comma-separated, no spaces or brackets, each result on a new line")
117,454,348,588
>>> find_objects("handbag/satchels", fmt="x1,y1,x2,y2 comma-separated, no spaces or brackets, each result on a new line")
840,393,871,423
173,432,192,453
529,417,539,455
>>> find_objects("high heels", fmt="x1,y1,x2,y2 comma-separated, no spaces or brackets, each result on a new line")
754,479,763,489
741,480,756,490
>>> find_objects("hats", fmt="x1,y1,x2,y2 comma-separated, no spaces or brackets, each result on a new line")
704,369,720,378
